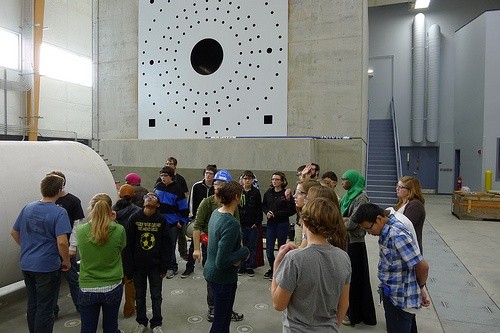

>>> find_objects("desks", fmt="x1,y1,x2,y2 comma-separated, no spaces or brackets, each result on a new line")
262,223,295,250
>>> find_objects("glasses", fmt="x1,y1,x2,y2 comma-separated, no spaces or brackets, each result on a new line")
295,189,306,194
243,178,252,180
361,220,375,231
271,178,281,181
397,184,409,189
159,174,169,177
296,171,302,176
310,168,316,171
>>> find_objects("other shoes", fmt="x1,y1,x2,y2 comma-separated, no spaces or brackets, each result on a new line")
133,324,164,333
342,315,352,326
237,268,255,277
207,309,215,322
230,312,244,321
180,269,194,278
54,312,58,319
263,269,274,281
166,269,179,279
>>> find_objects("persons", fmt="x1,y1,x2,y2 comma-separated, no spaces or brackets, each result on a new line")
355,177,432,333
111,157,190,318
9,174,73,332
270,198,352,333
179,164,297,281
202,180,250,333
46,171,85,318
75,193,127,333
292,162,378,325
125,191,176,333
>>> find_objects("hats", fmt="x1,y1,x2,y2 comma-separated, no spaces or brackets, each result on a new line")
118,184,135,199
125,173,141,185
165,157,176,164
213,169,232,183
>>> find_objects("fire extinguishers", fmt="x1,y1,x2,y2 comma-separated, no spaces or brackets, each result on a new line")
457,176,463,188
